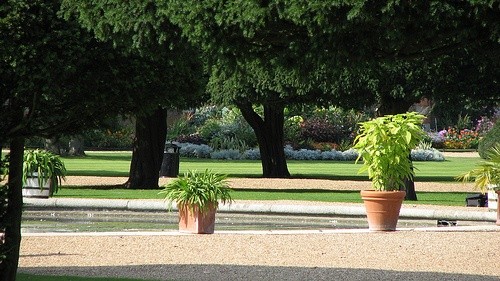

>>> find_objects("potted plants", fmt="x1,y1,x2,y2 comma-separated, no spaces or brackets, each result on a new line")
353,112,428,231
158,168,234,234
456,116,500,211
488,168,500,224
303,114,345,150
0,150,68,198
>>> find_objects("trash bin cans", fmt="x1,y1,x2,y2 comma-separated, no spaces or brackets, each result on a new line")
465,193,484,206
160,143,181,178
